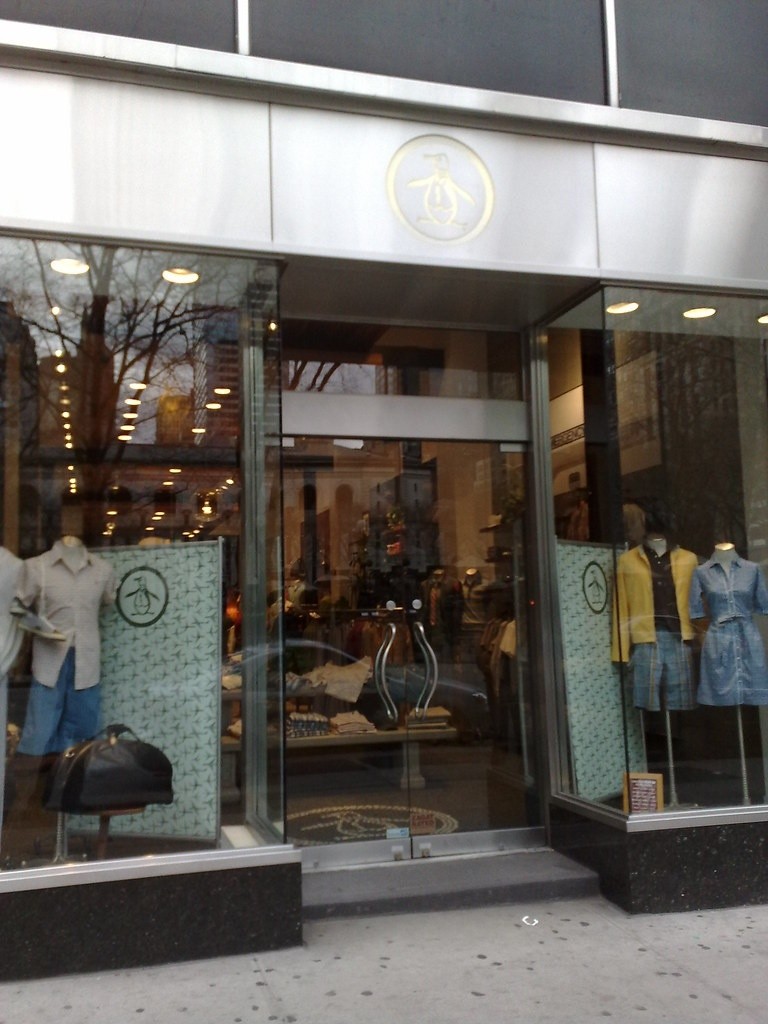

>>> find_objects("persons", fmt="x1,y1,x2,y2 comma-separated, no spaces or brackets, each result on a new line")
265,565,493,744
612,531,768,711
1,534,122,755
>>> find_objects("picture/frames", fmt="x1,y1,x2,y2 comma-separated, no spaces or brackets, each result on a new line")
622,772,664,813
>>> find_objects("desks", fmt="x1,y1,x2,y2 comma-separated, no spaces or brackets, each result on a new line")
50,807,146,861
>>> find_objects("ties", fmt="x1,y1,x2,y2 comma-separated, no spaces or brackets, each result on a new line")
430,583,437,627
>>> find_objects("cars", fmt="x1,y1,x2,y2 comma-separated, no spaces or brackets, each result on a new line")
143,634,490,785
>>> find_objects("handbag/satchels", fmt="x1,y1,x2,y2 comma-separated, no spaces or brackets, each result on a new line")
40,723,175,811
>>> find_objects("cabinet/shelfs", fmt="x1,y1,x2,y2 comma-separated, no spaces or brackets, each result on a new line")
372,521,440,613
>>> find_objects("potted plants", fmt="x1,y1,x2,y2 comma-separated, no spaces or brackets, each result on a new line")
386,502,415,529
